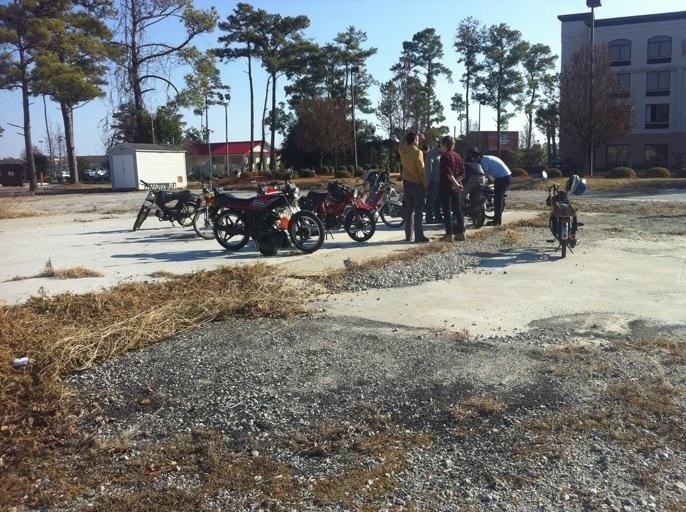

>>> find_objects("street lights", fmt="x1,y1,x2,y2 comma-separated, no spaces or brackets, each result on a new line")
204,89,227,193
587,0,601,175
216,92,232,177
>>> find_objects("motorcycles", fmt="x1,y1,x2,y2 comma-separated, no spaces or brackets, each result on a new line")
535,171,591,263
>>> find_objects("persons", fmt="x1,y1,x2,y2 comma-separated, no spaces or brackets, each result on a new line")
398,132,512,242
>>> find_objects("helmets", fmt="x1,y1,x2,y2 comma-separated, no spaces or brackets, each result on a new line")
573,177,587,196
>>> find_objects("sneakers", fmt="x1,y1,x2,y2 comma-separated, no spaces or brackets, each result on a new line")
415,235,431,242
439,233,454,243
454,232,466,241
405,234,413,241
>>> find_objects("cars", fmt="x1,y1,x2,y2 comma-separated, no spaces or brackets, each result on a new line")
58,161,108,181
